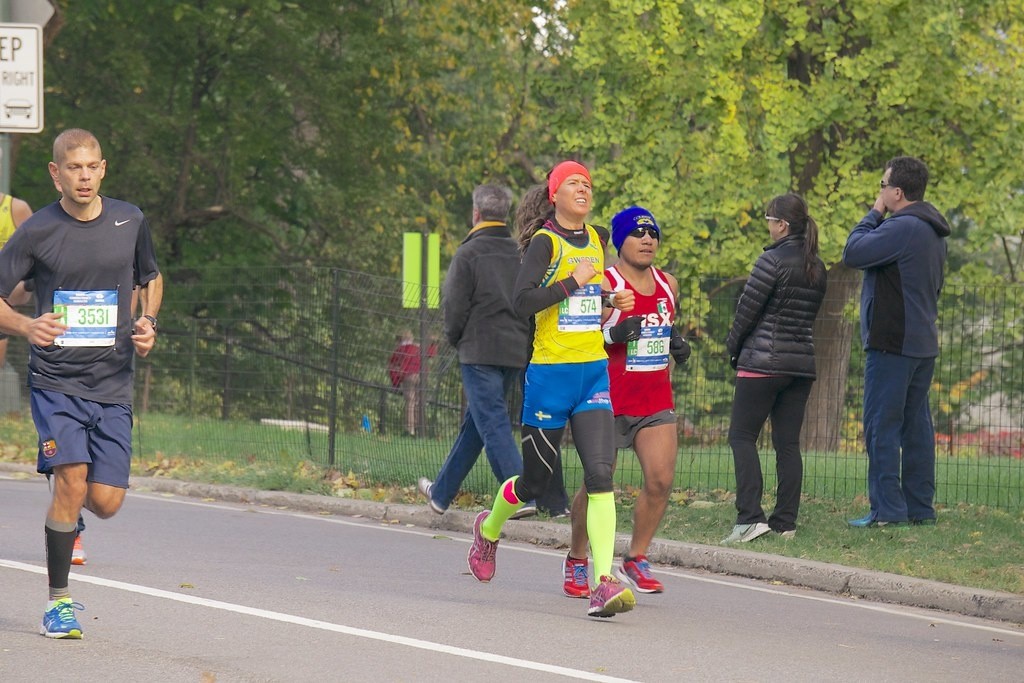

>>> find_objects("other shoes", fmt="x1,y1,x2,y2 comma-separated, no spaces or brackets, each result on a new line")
848,511,886,528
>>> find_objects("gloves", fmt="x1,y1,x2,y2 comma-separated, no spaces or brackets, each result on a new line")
610,316,644,343
667,336,691,366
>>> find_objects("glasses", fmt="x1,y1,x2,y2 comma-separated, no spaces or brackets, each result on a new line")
880,179,892,189
765,213,789,227
629,226,657,239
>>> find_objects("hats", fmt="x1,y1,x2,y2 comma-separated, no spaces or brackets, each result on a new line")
547,160,591,204
611,206,659,256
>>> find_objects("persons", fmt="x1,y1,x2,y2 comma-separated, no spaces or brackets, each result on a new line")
386,328,449,439
841,157,953,529
0,127,164,638
417,183,539,521
561,204,692,600
718,194,826,548
467,160,635,618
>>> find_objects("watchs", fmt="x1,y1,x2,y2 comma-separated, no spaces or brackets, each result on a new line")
144,315,158,332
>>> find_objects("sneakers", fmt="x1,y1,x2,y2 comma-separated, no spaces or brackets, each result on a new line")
468,509,500,586
621,553,662,592
561,549,590,598
780,529,797,540
509,498,537,519
586,573,635,618
720,519,771,546
70,535,85,566
417,477,446,515
38,598,85,639
